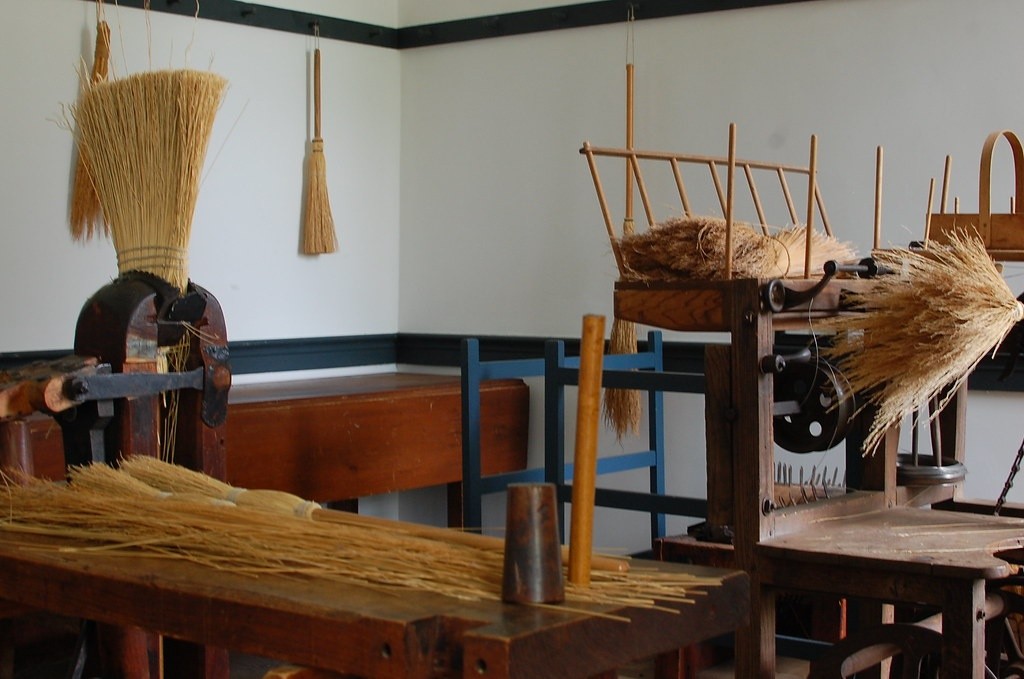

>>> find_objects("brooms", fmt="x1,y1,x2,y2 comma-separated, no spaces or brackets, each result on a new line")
68,19,112,250
301,49,341,256
60,453,630,583
808,217,1024,458
44,0,243,679
596,62,643,448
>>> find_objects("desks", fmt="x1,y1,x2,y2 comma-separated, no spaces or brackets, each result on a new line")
0,372,531,679
0,503,749,679
